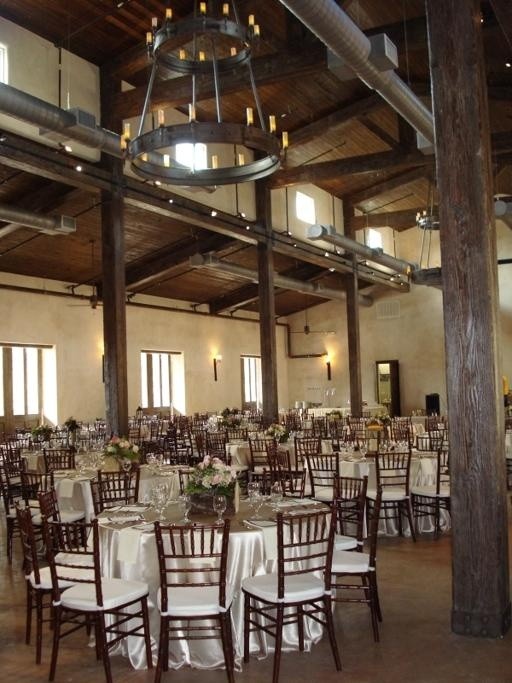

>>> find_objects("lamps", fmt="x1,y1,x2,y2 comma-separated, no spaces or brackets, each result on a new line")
407,153,443,285
118,1,290,186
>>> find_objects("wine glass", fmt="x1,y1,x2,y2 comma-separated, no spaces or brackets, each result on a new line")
14,405,447,477
213,482,282,526
151,484,169,505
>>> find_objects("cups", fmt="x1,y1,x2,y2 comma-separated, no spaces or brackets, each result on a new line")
138,507,156,521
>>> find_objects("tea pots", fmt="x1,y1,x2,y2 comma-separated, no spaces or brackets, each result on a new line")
158,501,191,518
